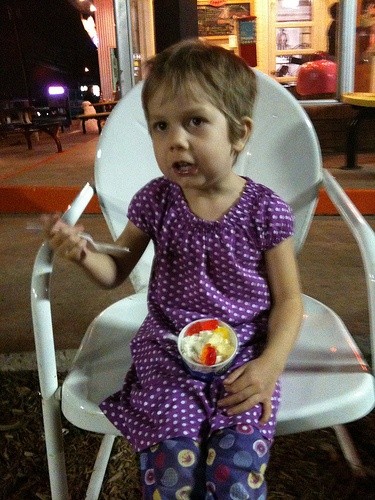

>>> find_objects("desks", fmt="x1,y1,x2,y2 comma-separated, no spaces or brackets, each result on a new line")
89,100,118,111
341,92,374,171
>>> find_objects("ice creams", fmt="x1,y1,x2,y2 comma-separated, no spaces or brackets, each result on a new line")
180,319,235,372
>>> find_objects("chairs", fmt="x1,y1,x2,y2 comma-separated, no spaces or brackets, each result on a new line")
30,67,375,500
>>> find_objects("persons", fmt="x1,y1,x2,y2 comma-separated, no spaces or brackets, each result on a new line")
38,39,304,500
327,2,337,55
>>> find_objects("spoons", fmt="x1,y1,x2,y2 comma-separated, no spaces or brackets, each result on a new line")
27,228,130,256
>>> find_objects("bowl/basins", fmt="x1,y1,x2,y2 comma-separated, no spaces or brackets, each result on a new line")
177,318,238,374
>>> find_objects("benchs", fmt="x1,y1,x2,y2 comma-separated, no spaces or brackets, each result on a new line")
75,113,109,135
31,117,67,134
14,123,64,153
